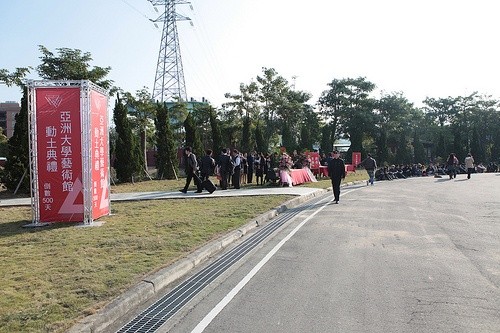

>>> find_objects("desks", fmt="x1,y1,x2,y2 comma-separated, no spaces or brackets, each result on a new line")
280,165,354,187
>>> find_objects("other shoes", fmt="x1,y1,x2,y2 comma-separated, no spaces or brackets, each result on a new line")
261,183,263,185
194,190,202,193
331,199,336,202
370,183,374,185
179,189,187,193
334,200,340,204
454,174,456,179
449,177,453,179
366,180,369,186
257,184,259,185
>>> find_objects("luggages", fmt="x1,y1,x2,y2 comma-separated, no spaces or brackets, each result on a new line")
194,172,217,194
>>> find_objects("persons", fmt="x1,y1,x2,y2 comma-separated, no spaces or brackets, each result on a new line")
375,161,498,182
327,150,346,204
447,154,455,179
179,147,282,194
453,153,458,178
465,154,474,179
354,153,377,186
